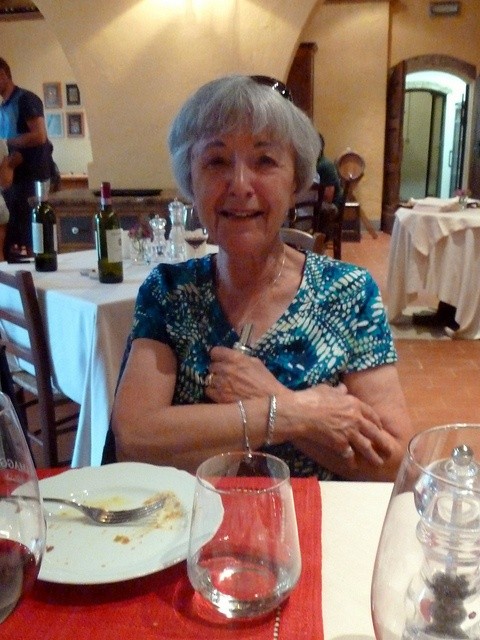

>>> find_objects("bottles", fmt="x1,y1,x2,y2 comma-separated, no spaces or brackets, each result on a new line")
402,444,479,640
149,214,168,267
31,180,58,273
93,182,125,283
164,197,187,263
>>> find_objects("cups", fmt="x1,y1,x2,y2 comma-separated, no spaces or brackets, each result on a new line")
186,451,302,619
0,390,45,624
369,423,480,640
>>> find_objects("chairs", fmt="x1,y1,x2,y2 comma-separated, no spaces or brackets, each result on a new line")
324,175,351,260
289,183,327,232
278,228,327,255
0,271,81,467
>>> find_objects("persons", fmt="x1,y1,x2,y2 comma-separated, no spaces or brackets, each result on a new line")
49,142,61,193
111,73,416,486
0,56,55,264
0,138,20,263
312,132,342,255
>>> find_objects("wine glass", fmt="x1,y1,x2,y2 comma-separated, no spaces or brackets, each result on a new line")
181,204,209,257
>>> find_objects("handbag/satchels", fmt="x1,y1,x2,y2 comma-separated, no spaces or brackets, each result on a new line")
32,138,53,183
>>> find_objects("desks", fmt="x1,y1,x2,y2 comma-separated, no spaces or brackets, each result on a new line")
28,188,195,253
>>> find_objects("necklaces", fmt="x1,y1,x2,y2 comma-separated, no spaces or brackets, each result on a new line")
211,252,286,327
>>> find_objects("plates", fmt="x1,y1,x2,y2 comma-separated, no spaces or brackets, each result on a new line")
1,461,224,584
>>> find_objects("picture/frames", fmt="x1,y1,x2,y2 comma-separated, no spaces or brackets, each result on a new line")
45,113,64,137
66,84,80,105
43,82,63,109
67,112,84,138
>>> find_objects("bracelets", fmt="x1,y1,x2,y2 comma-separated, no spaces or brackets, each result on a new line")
238,400,252,464
265,395,277,450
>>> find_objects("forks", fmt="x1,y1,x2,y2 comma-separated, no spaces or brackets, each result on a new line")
1,494,166,525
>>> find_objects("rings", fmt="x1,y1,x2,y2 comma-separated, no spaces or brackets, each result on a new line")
339,445,357,461
205,373,213,387
